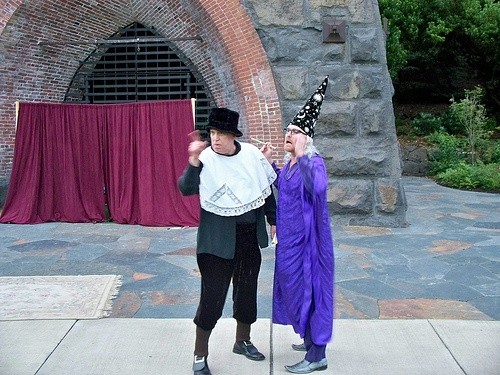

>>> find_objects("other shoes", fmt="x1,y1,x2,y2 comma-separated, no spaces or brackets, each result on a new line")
292,343,307,351
285,358,327,374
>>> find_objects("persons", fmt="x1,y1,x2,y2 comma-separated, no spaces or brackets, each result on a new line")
176,107,277,375
260,121,335,374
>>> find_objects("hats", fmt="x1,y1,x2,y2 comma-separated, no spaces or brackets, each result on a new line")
204,107,244,138
289,75,329,138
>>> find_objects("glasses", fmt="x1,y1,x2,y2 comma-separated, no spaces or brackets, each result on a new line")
283,127,306,136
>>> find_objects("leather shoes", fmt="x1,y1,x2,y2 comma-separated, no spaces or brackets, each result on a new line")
192,355,212,375
232,341,265,361
